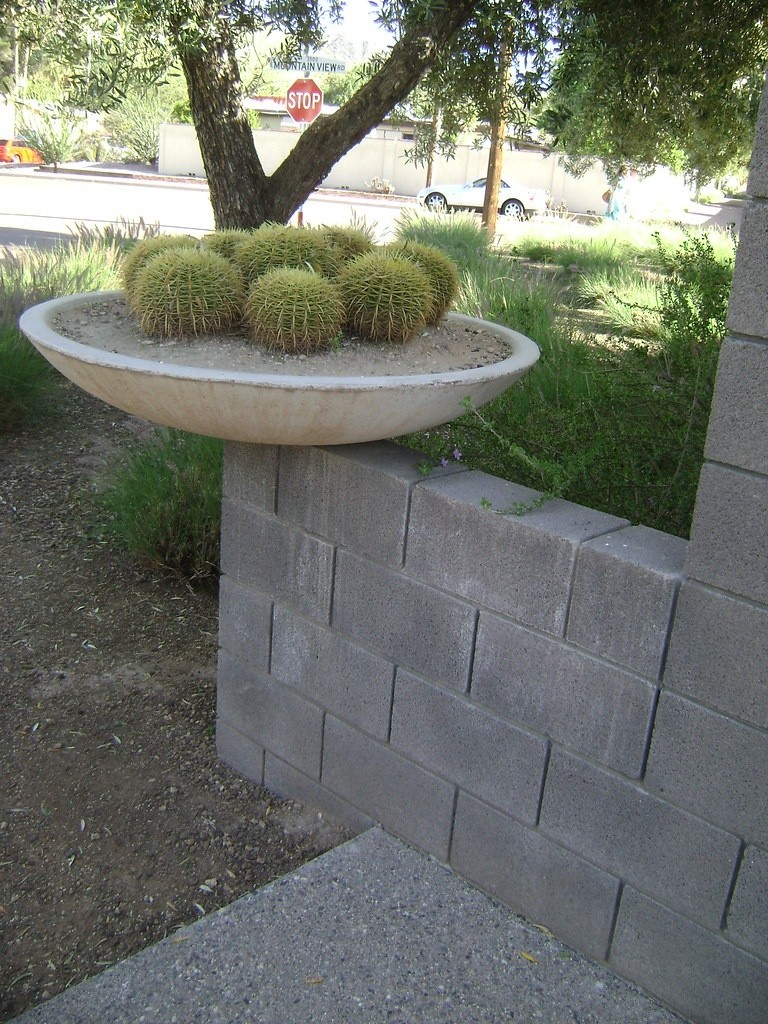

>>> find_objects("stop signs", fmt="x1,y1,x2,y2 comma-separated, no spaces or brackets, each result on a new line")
285,79,325,125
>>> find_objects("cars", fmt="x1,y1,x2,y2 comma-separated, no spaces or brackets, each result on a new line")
416,175,547,219
0,139,46,163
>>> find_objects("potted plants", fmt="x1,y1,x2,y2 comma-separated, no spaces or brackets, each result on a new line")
17,217,540,444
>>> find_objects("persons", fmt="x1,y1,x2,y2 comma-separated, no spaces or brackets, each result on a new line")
606,176,623,219
